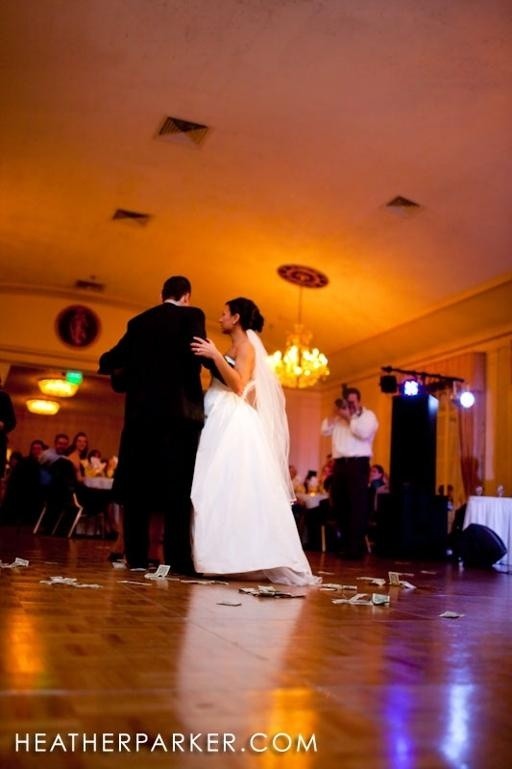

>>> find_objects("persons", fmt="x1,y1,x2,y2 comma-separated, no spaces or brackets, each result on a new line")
319,382,379,558
1,388,16,481
1,434,510,566
94,275,235,577
190,297,322,588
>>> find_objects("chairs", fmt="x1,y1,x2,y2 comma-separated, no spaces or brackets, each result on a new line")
291,485,392,556
29,474,114,542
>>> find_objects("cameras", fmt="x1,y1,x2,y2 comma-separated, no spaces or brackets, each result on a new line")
335,383,351,410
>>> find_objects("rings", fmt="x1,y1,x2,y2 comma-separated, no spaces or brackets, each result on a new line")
197,347,200,351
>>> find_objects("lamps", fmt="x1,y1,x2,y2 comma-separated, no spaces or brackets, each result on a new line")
379,373,477,415
267,279,331,389
24,376,80,419
